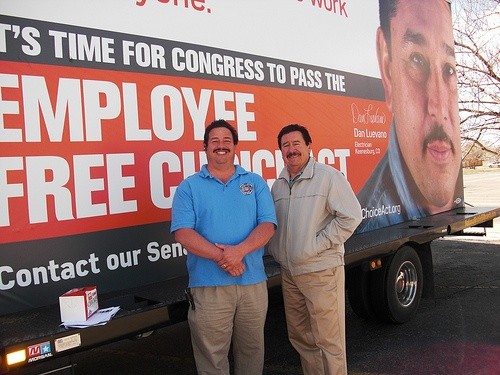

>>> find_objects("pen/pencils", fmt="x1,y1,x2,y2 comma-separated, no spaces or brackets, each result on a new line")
99,309,112,313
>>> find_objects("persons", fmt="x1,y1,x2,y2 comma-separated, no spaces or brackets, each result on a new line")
353,0,464,236
170,120,278,375
264,124,363,375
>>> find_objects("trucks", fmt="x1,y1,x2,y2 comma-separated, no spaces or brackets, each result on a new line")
0,0,499,375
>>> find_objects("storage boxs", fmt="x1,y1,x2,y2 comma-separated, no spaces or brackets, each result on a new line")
59,286,98,322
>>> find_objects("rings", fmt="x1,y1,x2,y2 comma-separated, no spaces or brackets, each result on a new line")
223,264,226,267
